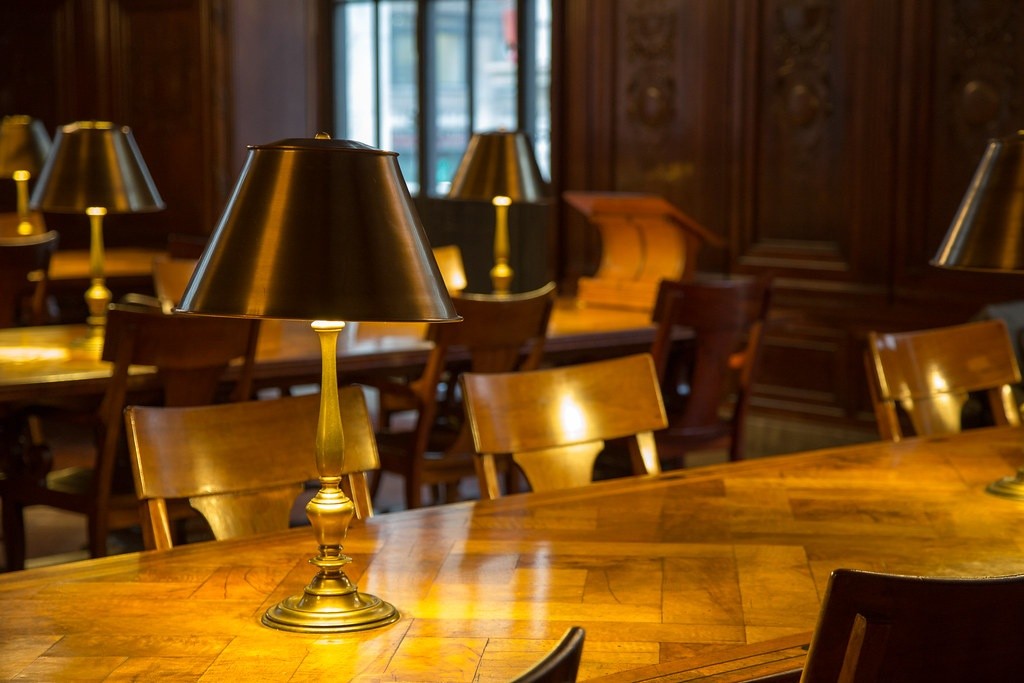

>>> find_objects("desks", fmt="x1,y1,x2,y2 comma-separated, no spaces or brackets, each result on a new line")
23,250,175,506
0,300,696,574
0,424,1024,683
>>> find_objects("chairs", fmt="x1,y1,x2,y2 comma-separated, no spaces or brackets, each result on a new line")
0,213,1024,683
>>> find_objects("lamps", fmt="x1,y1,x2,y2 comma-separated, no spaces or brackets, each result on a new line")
172,134,463,631
0,115,53,235
446,131,553,296
927,129,1024,500
25,121,164,362
